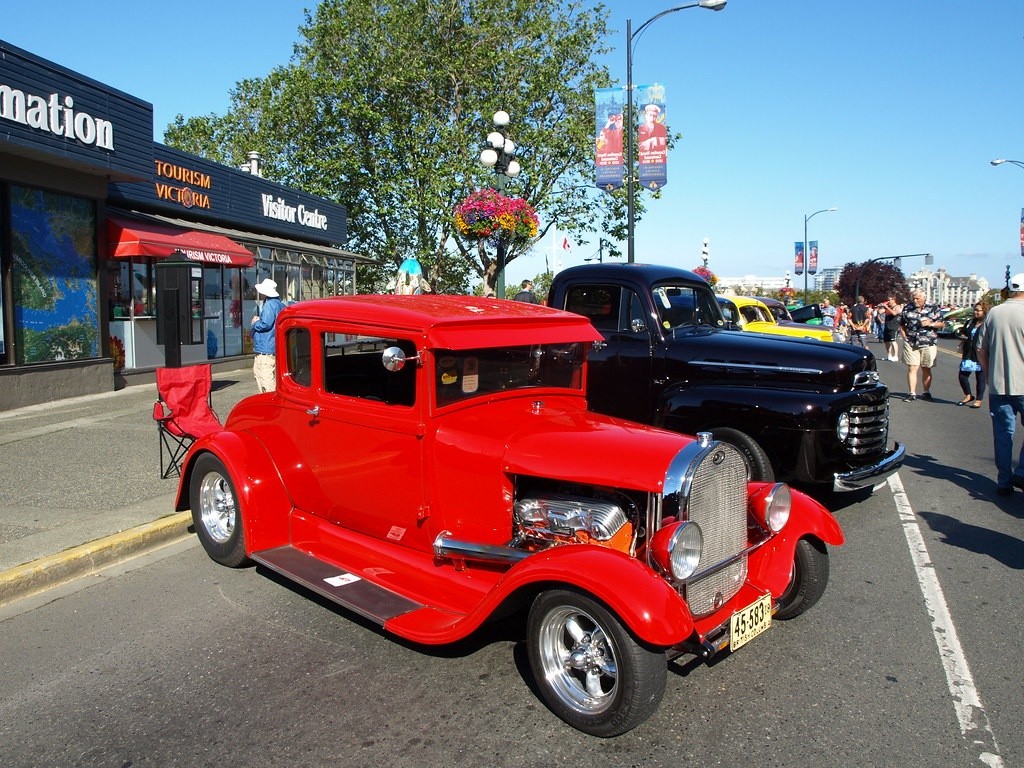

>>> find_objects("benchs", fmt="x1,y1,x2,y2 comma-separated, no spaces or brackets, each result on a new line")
323,349,415,402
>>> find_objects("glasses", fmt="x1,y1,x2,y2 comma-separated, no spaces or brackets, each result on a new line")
528,284,532,286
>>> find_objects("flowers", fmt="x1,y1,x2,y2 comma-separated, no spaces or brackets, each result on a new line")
454,189,539,248
692,266,718,289
777,288,796,299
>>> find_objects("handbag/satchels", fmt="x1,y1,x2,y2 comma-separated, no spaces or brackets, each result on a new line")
960,359,982,372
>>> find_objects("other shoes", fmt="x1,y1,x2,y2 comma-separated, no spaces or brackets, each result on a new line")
1011,474,1024,492
957,395,975,406
904,393,918,403
881,355,893,361
997,486,1014,496
921,392,933,402
891,356,899,362
973,400,982,408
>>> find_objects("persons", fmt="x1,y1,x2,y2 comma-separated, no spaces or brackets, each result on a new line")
899,291,945,401
956,301,990,408
637,104,667,164
513,279,537,305
595,113,623,165
818,291,961,363
975,272,1024,496
810,247,816,265
250,278,287,391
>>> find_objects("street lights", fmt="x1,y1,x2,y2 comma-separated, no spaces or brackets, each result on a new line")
701,238,709,266
785,271,792,288
481,111,521,298
805,208,838,305
627,0,728,263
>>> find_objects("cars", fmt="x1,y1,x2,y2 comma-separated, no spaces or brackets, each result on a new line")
650,291,834,343
175,292,844,738
546,261,905,493
928,307,973,339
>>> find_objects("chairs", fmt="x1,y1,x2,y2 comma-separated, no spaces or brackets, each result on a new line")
153,363,224,480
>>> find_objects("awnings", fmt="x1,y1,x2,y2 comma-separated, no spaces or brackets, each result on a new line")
107,216,254,268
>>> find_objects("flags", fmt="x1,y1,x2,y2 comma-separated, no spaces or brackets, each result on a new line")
562,238,572,254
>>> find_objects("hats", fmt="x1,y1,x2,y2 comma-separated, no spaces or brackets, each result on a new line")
1008,273,1024,291
255,278,281,299
